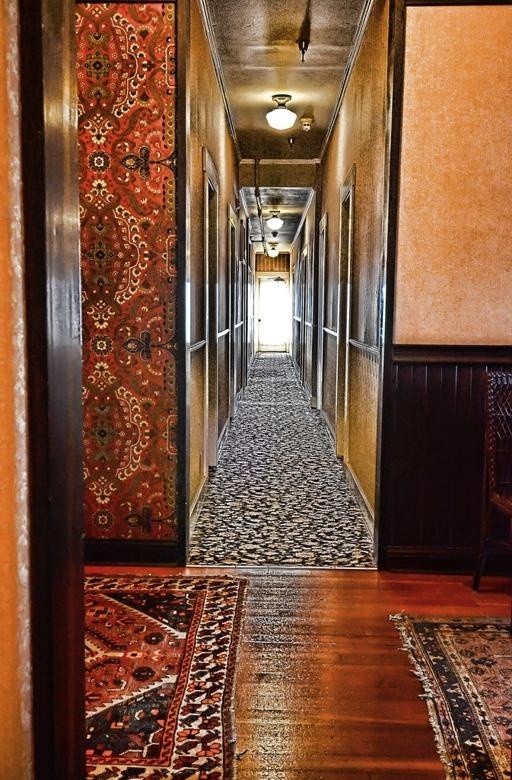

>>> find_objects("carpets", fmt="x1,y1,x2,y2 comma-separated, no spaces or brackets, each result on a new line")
77,570,247,780
390,605,512,780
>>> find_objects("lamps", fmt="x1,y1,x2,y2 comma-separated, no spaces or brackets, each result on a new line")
265,90,297,131
265,210,285,230
266,238,280,258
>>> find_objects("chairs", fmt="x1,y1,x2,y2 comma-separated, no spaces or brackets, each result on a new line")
472,368,512,592
